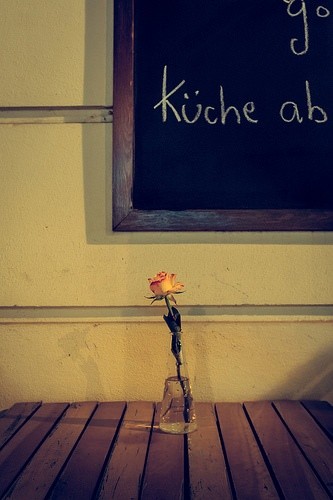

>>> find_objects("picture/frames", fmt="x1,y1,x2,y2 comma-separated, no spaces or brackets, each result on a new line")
107,0,333,234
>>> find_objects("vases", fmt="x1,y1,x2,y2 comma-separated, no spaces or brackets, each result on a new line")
155,331,200,434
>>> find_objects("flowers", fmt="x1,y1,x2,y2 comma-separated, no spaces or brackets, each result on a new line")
143,271,197,426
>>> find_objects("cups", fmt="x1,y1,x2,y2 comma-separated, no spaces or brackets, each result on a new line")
159,376,199,436
